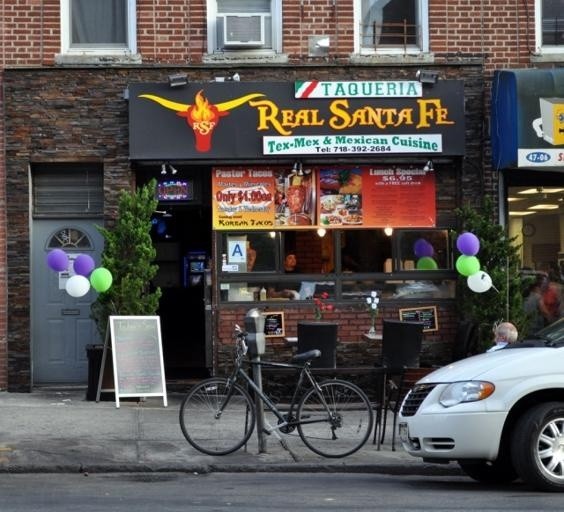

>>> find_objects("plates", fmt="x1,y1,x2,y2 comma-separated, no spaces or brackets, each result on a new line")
287,215,311,226
342,216,363,224
322,215,343,225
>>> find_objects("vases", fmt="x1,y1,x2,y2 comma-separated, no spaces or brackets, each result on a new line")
315,311,321,321
369,324,378,336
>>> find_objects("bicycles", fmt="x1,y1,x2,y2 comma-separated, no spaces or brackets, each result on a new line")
179,324,374,459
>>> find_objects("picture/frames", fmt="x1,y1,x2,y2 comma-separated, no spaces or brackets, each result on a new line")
399,306,438,332
262,311,285,338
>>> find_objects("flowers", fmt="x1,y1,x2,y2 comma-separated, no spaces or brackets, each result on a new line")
312,292,334,311
366,292,380,323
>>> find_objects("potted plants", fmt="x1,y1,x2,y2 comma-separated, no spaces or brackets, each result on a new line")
83,178,162,401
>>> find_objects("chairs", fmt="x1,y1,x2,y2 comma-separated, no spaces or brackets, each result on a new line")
381,367,440,451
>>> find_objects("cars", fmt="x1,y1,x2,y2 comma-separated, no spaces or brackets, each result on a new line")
397,319,564,492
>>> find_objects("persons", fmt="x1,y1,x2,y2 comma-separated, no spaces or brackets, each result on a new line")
522,278,563,335
246,248,257,287
486,322,518,353
266,251,301,297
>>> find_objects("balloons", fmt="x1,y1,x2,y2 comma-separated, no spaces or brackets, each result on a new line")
457,232,480,256
65,274,90,298
73,253,94,278
455,254,480,276
90,267,113,292
417,256,438,270
467,271,492,293
46,248,69,272
414,239,434,257
